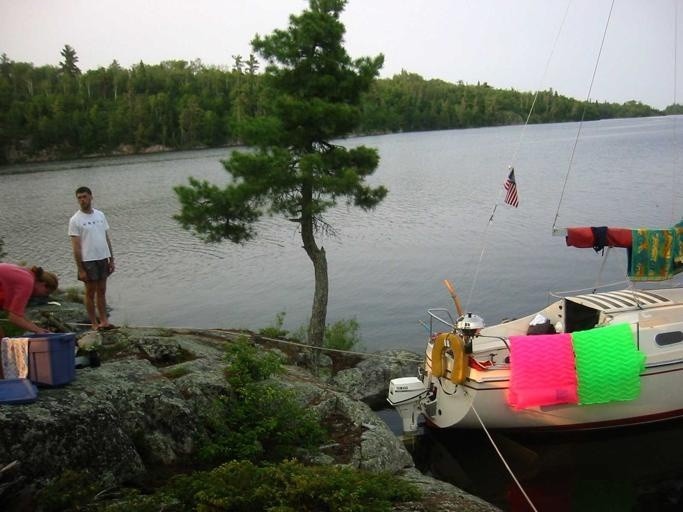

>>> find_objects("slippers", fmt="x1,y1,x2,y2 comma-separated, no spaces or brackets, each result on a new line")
97,323,121,329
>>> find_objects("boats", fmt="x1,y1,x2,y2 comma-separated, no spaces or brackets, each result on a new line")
385,269,682,437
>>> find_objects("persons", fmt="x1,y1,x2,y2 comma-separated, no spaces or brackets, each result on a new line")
0,263,59,379
68,186,115,331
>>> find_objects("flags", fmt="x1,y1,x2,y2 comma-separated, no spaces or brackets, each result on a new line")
502,168,521,208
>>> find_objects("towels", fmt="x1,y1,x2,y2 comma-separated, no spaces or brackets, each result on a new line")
627,220,683,281
1,337,31,381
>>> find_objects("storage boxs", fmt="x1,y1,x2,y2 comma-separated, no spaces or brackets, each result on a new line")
0,332,77,386
